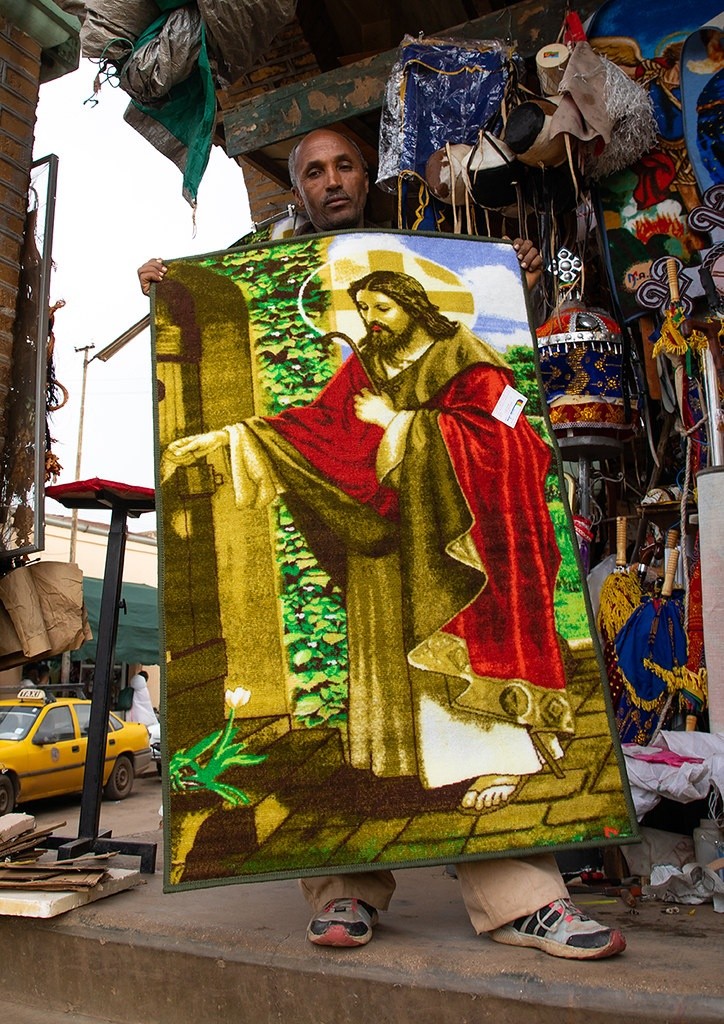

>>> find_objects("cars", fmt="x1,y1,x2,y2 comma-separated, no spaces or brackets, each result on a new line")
0,682,154,816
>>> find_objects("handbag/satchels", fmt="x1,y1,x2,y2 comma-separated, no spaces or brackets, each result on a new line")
117,686,135,711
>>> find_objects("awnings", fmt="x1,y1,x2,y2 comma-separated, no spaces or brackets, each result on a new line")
45,576,161,670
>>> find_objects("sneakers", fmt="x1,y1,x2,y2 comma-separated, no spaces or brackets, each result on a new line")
304,898,379,946
490,898,627,960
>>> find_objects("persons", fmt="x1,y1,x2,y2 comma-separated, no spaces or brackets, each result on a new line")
20,661,50,686
138,128,626,958
126,671,160,754
